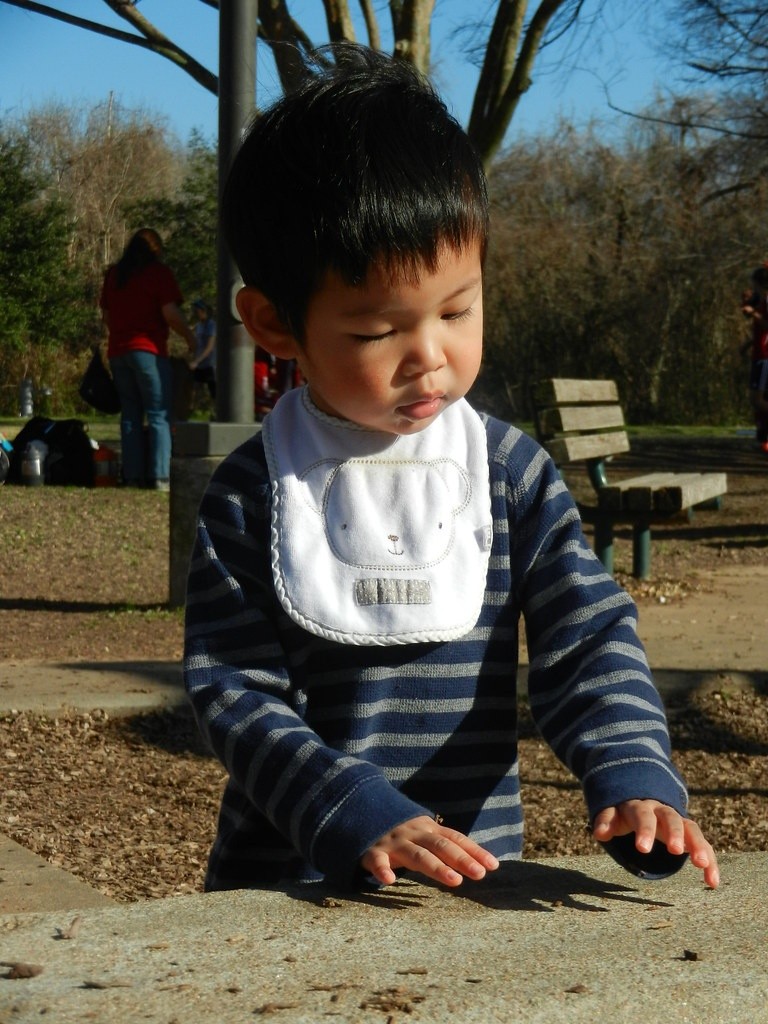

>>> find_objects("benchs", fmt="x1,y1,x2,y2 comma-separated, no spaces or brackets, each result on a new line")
540,378,728,582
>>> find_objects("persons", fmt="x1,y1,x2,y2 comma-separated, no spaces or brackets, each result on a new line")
740,267,768,451
254,343,282,418
178,301,215,419
185,41,723,895
99,231,194,495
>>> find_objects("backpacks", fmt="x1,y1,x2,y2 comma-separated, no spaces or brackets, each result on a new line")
9,416,47,485
42,419,96,488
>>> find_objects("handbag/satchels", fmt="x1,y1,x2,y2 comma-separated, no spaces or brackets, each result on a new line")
78,351,123,415
